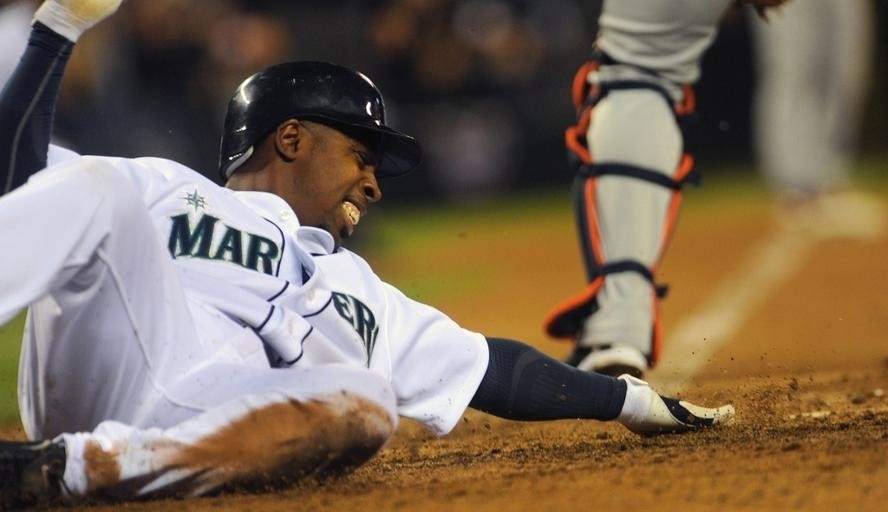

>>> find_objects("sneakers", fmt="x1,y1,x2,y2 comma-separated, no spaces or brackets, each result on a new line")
0,438,67,511
563,344,648,378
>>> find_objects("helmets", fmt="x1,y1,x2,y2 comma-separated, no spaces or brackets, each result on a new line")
218,61,423,183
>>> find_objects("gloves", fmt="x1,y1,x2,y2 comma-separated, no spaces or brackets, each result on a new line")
616,373,735,433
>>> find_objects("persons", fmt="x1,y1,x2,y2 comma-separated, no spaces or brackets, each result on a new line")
544,0,737,380
0,1,736,511
